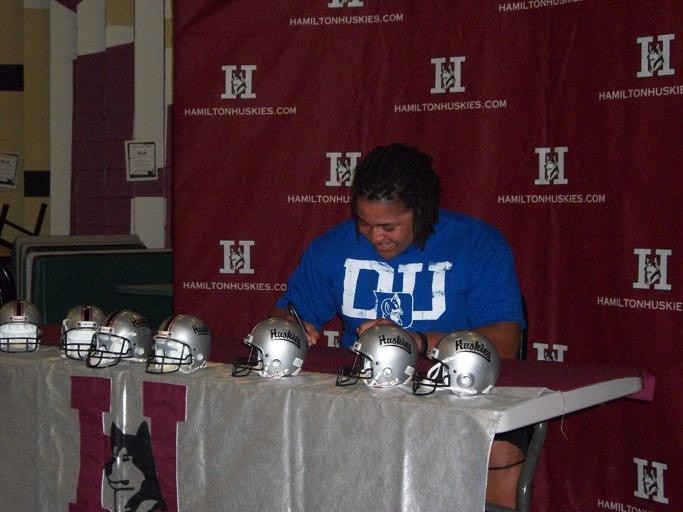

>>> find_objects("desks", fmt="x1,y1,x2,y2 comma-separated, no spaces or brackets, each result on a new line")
1,321,646,507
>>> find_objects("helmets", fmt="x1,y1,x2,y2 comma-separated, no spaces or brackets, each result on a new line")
96,309,152,363
352,322,418,390
0,299,44,352
431,328,500,397
152,313,211,372
59,304,108,352
245,316,309,378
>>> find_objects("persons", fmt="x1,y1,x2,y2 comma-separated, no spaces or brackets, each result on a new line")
270,141,532,512
